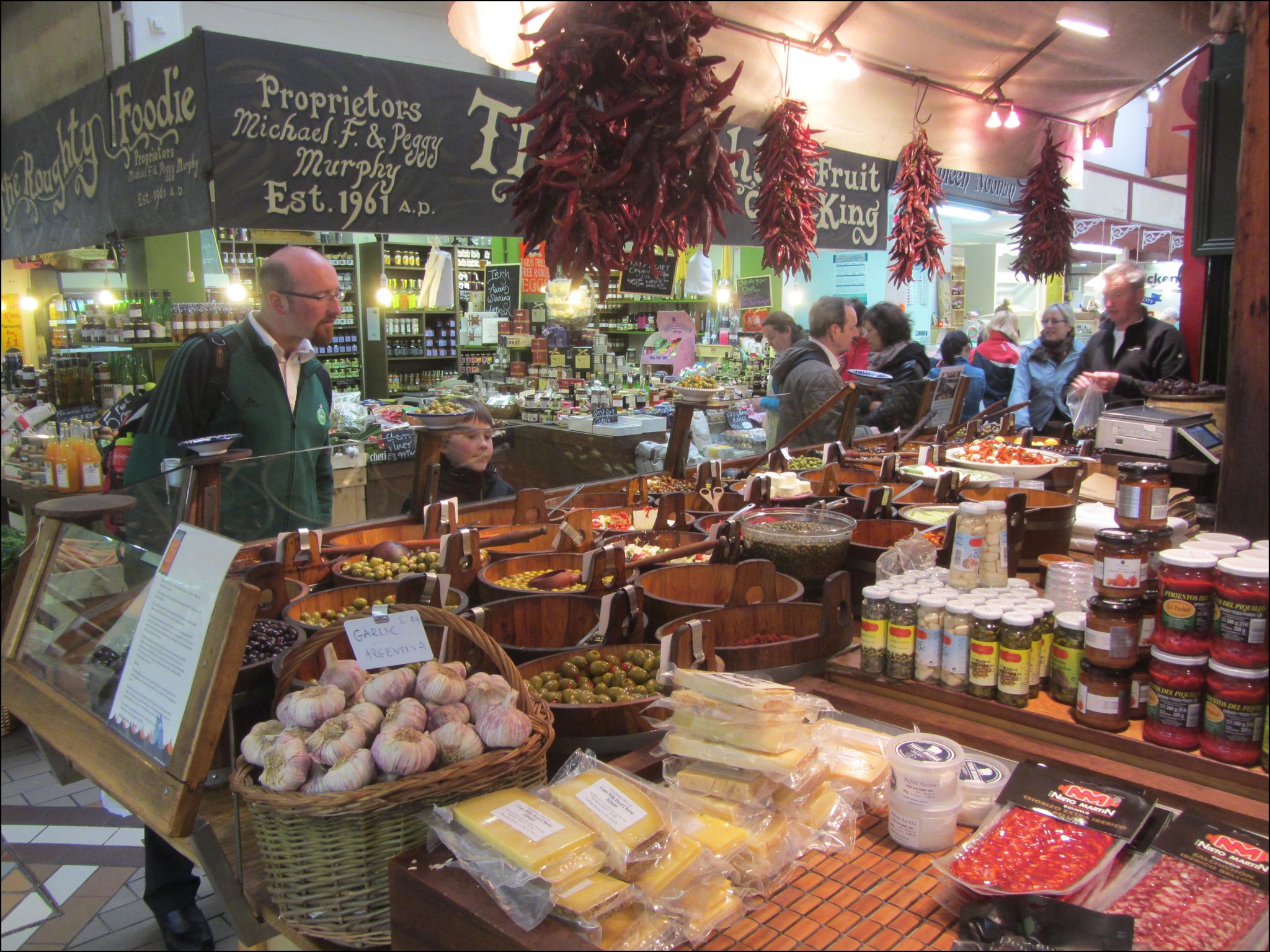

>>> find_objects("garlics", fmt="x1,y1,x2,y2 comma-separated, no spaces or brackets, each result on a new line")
240,643,532,796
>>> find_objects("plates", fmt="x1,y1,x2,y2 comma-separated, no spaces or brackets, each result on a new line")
406,408,473,430
178,433,243,456
849,367,892,386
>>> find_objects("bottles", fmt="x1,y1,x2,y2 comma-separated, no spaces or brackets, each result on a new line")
637,300,740,347
670,381,724,403
44,286,175,494
597,370,650,404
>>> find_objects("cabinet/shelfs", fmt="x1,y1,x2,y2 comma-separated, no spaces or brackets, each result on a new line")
359,240,460,401
216,239,363,402
584,272,713,336
949,264,966,327
455,246,493,314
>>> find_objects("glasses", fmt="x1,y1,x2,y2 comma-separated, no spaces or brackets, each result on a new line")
276,290,347,302
1041,318,1068,325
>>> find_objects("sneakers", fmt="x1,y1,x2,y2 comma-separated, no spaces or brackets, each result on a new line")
153,899,216,952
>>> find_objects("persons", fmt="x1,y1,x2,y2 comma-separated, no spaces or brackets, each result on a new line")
400,396,520,513
752,260,1198,453
123,245,347,951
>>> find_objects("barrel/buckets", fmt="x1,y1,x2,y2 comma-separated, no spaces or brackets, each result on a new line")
196,420,1102,770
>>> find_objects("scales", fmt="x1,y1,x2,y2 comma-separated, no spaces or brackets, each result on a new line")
1094,399,1226,465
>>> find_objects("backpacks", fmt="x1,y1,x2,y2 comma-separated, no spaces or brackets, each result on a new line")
100,331,230,542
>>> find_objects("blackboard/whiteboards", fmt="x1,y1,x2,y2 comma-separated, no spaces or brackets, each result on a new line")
617,250,679,297
725,409,753,431
592,407,618,425
736,275,773,310
381,429,417,461
484,263,521,321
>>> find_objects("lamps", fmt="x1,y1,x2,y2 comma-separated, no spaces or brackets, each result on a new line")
826,33,858,83
1054,0,1112,38
789,281,803,306
716,278,729,305
986,85,1021,129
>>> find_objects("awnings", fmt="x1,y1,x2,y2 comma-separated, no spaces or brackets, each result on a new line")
444,1,1223,178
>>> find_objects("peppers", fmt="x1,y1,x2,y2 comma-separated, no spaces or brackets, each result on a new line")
752,87,828,282
883,128,948,290
498,0,745,304
1007,126,1076,286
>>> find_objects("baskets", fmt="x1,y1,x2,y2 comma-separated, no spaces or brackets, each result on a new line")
229,600,551,944
